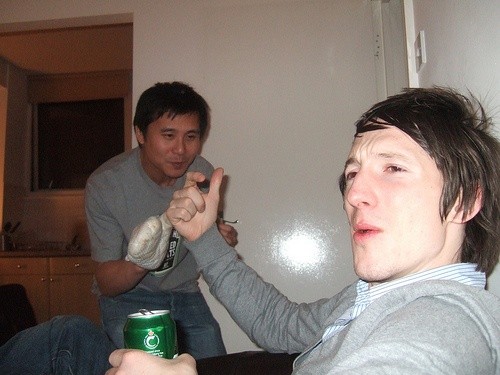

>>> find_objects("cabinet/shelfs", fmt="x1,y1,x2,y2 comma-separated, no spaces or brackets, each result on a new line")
0,256,100,326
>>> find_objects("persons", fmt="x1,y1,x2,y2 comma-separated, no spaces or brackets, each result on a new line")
82,80,239,361
0,83,500,375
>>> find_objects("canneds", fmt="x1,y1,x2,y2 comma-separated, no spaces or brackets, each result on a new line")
124,308,179,359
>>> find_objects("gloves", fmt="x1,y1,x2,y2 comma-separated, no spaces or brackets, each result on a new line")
125,210,173,270
